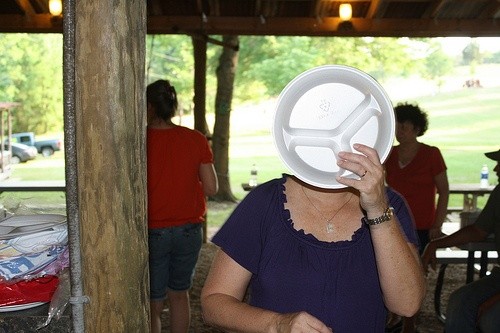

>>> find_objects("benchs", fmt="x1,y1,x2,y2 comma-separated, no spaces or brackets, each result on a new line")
434,249,498,325
447,207,479,212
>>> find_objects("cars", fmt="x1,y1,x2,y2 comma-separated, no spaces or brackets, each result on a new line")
0,141,38,165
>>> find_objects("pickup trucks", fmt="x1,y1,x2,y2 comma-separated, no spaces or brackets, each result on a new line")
4,131,61,157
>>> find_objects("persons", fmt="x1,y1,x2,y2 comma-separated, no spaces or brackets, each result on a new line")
146,79,219,333
200,143,427,333
422,148,500,333
383,101,449,333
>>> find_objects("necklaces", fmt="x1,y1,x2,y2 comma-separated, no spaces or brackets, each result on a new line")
302,184,354,232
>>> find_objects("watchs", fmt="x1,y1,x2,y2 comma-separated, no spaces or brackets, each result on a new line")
364,208,393,225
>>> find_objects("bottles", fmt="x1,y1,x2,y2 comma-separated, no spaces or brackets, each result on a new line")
249,163,257,186
480,164,488,188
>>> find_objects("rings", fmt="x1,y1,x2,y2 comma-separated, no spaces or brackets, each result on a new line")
361,170,367,177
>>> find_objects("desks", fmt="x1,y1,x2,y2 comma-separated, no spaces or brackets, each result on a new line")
440,221,498,284
437,184,495,210
